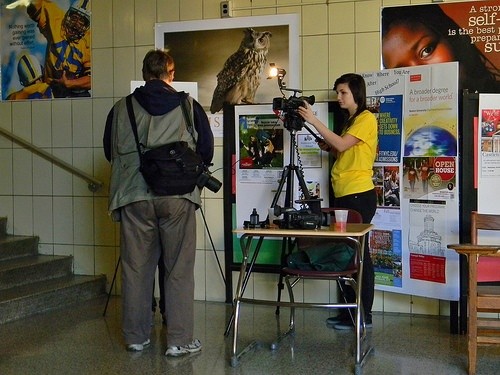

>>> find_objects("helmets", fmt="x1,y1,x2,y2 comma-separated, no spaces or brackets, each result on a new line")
17,55,43,87
59,0,92,41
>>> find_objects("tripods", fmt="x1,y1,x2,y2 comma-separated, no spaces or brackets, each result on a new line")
224,129,364,339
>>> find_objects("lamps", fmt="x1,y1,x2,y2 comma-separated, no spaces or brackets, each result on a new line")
266,62,286,89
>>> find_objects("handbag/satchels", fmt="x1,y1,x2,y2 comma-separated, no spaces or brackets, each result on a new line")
291,240,357,272
140,141,205,196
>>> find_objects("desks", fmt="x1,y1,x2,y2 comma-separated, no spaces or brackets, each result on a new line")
230,223,374,375
448,244,500,375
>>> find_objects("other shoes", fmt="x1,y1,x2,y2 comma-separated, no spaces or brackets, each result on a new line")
165,337,202,356
127,339,150,351
326,316,373,329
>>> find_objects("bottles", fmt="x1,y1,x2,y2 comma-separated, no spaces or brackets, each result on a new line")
250,208,259,225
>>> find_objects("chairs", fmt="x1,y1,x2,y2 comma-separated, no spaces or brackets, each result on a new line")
472,210,500,348
271,208,366,353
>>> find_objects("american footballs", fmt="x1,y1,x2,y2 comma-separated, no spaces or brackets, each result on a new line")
53,69,78,80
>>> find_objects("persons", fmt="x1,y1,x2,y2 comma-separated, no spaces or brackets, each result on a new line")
408,167,431,195
297,73,378,330
382,4,499,93
102,49,214,356
8,0,90,100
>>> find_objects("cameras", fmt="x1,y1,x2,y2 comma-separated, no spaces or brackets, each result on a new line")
195,173,222,193
285,211,331,230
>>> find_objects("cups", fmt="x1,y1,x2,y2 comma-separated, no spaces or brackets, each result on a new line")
268,208,280,226
334,209,348,228
